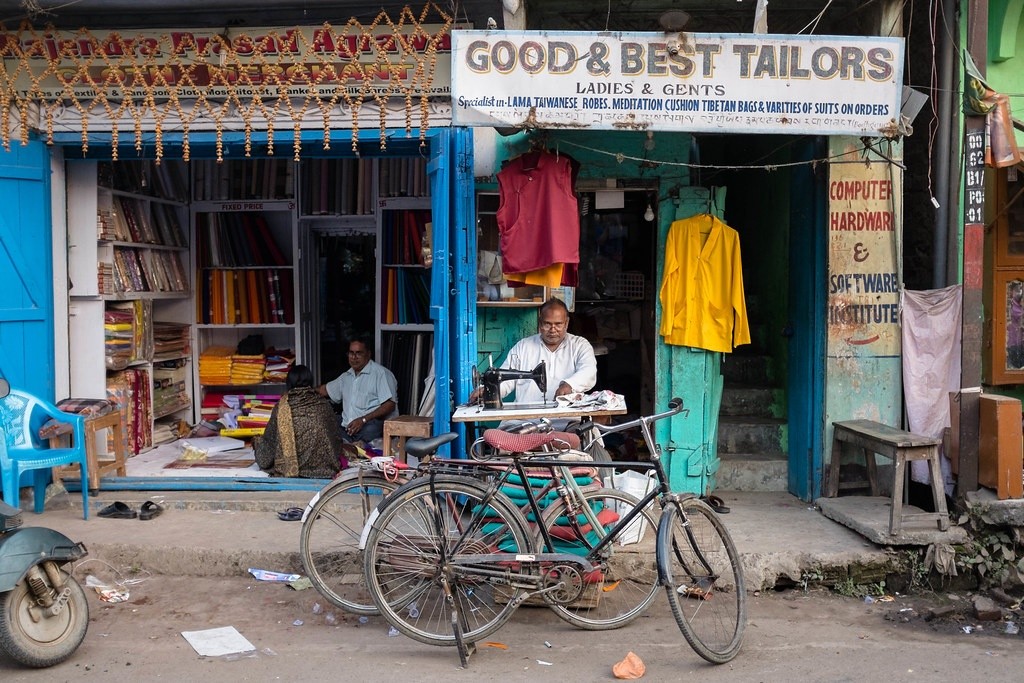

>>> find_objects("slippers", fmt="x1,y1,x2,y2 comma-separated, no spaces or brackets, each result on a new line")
278,507,321,521
97,502,138,519
140,501,162,519
701,495,731,513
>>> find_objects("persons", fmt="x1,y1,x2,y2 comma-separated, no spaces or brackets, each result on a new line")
590,237,643,413
1007,281,1024,368
316,336,399,445
469,295,604,448
251,365,343,480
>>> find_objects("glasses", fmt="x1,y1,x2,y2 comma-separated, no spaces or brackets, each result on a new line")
540,319,567,331
349,352,369,359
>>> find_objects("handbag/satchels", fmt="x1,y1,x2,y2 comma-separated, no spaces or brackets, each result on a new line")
603,470,657,547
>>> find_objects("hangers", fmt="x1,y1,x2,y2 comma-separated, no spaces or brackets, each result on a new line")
494,130,582,177
647,166,740,239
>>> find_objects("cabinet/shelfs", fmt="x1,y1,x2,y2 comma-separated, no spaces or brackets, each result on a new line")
64,155,435,460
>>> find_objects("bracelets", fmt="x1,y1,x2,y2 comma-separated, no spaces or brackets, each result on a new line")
362,417,367,423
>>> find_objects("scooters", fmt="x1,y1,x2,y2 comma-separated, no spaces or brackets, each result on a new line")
0,501,92,671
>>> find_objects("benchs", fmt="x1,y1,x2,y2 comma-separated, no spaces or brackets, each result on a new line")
826,420,950,536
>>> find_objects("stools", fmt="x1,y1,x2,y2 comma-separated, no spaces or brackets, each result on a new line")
49,410,127,497
382,415,434,499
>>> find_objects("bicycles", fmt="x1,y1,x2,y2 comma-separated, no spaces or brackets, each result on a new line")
299,430,665,617
357,398,749,670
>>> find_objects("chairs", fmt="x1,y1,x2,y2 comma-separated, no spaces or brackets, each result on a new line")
0,388,90,520
